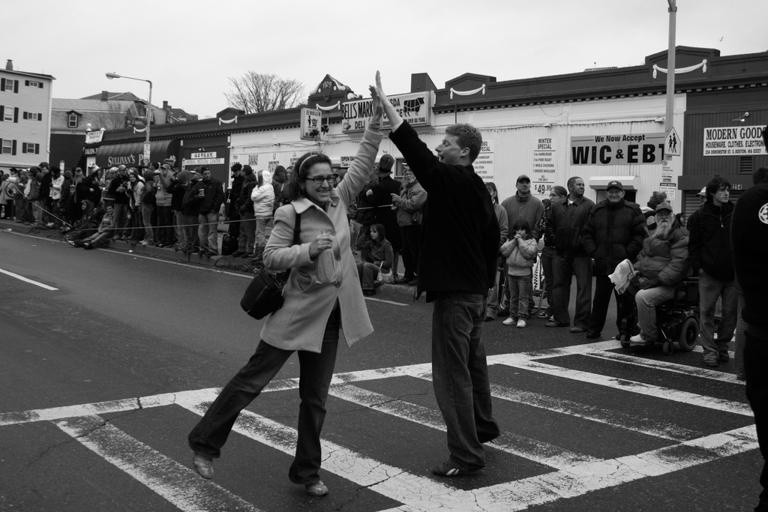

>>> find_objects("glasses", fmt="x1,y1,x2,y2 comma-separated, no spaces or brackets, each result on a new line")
305,173,337,184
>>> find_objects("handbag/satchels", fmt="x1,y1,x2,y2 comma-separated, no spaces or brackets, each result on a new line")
376,260,395,283
240,268,289,321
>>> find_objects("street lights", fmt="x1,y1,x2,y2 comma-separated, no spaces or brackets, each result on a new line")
100,70,155,168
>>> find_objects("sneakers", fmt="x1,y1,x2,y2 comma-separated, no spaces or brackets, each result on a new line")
502,317,517,325
305,479,329,497
629,334,650,343
516,319,526,327
192,452,215,478
537,310,622,341
702,343,730,367
0,215,265,263
397,273,419,286
431,461,485,478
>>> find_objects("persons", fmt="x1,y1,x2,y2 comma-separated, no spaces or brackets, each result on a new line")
375,70,500,478
731,126,767,511
190,86,383,498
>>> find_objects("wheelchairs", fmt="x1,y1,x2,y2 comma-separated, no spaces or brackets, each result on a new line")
609,255,702,357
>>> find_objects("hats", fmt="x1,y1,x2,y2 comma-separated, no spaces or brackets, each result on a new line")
606,180,624,192
655,202,673,214
1,162,257,180
516,174,531,183
